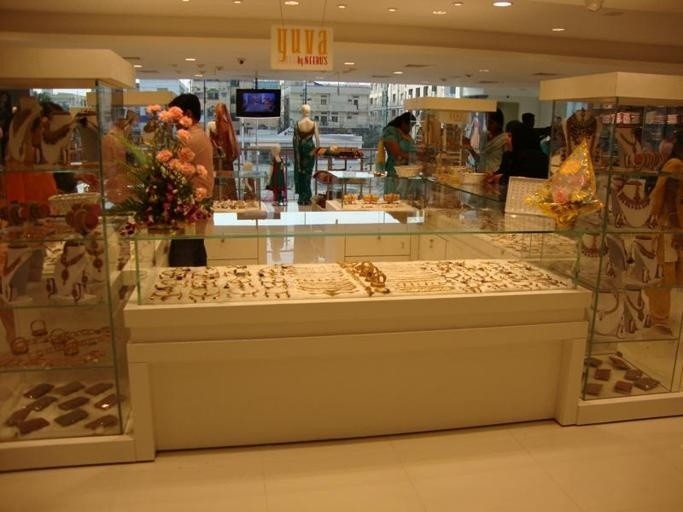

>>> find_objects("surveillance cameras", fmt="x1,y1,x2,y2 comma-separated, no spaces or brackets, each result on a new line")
238,58,245,64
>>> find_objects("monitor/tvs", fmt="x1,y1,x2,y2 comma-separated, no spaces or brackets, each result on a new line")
235,88,281,119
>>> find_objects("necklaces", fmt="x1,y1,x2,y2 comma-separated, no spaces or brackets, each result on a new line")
590,292,620,322
616,180,650,211
2,250,27,275
627,293,646,321
563,110,599,157
84,244,107,271
148,255,567,305
616,132,641,166
58,251,85,284
578,235,609,258
632,240,657,261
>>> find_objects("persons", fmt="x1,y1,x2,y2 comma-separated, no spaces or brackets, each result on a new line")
374,112,423,200
265,143,287,206
292,105,319,206
502,116,521,152
660,128,678,164
643,131,681,288
521,110,553,142
658,126,675,156
164,91,214,267
0,94,101,196
204,101,240,200
461,104,509,175
242,162,257,201
494,126,549,194
468,113,482,150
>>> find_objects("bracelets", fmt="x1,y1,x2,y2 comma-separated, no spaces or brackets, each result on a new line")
6,318,106,366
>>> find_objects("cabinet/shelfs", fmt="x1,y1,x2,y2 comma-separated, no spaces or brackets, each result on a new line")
1,48,683,471
